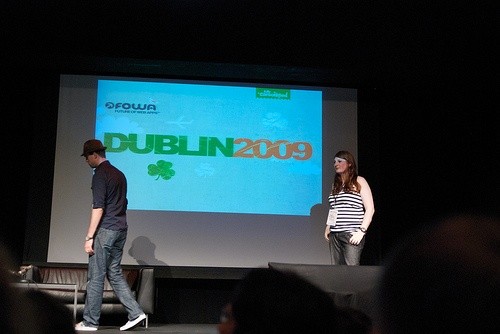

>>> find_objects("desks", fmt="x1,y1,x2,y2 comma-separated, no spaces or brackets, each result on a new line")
1,282,77,325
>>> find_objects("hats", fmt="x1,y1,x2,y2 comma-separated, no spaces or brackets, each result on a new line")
80,139,109,158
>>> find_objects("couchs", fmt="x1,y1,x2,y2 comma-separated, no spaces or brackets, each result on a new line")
18,265,154,328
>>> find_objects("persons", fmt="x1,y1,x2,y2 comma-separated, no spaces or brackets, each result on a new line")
325,150,375,266
75,140,148,332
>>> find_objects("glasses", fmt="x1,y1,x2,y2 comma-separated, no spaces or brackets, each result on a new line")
219,308,231,325
333,159,347,165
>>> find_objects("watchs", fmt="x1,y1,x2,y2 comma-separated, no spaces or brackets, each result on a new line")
358,226,368,233
84,236,94,240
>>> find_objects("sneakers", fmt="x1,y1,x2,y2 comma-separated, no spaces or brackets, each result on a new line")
74,320,98,332
119,312,147,332
